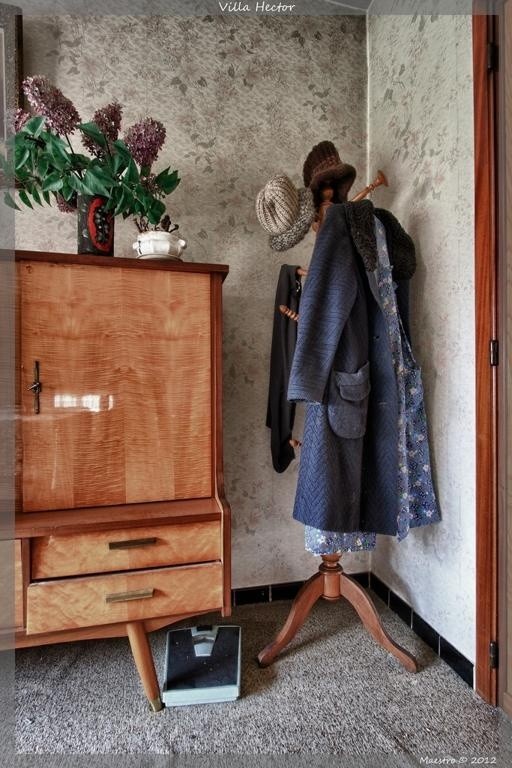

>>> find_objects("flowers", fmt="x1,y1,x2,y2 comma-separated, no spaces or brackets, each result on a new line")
2,75,183,226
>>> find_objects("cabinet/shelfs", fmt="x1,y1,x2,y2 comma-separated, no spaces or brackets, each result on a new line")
1,248,234,713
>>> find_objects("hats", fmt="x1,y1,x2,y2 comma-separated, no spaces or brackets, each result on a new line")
255,174,317,252
304,141,357,207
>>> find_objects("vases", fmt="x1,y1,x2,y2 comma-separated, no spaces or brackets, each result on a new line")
76,195,114,257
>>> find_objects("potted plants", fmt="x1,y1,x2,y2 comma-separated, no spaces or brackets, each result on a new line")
132,212,187,259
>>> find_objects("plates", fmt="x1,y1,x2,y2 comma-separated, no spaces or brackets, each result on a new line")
137,253,182,260
